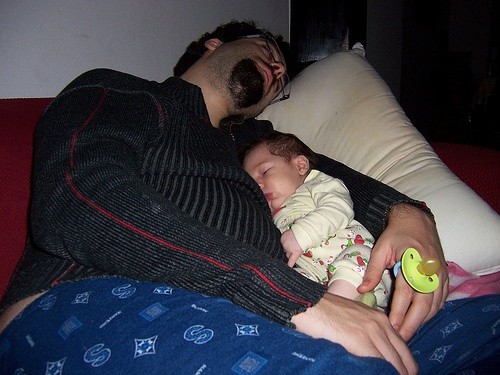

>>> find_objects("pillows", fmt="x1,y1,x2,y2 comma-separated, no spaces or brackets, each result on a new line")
265,43,500,281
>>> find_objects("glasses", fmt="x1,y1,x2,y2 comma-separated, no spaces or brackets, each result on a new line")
233,33,292,106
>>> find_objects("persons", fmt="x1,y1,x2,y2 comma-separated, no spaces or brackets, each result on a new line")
2,19,499,374
237,130,394,318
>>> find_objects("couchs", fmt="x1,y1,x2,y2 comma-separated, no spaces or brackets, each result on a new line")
0,96,500,301
